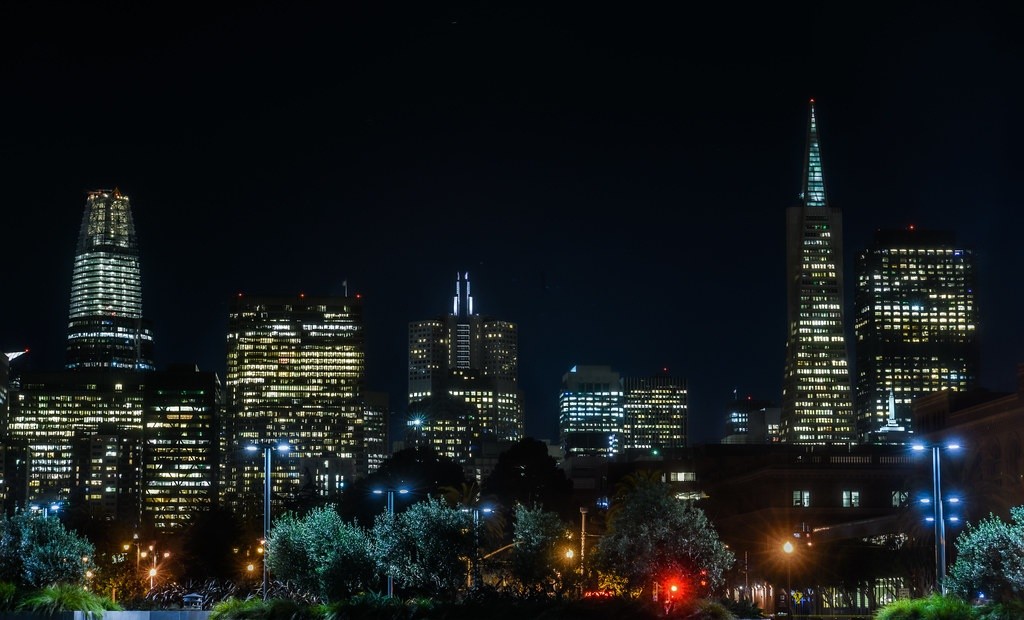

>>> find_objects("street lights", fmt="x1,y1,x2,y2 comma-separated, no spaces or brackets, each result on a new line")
244,442,292,596
911,443,963,596
29,503,60,521
458,506,493,587
782,541,795,619
372,488,410,600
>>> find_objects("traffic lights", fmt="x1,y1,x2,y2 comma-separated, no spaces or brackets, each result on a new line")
699,569,708,587
